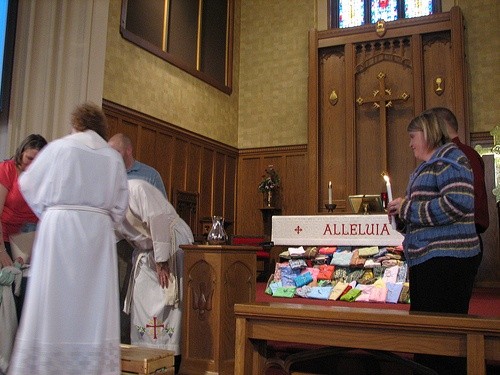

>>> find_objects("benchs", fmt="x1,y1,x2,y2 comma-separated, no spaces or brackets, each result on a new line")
121,347,175,374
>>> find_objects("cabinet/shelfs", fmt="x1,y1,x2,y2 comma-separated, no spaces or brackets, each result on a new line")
178,245,262,375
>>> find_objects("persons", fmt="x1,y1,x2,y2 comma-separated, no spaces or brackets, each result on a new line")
116,179,194,357
386,112,482,315
108,133,169,201
426,108,490,234
0,134,48,267
6,102,130,375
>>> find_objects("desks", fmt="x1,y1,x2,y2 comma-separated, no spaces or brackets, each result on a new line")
233,302,500,375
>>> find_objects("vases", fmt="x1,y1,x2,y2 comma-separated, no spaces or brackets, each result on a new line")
207,216,228,245
267,191,273,207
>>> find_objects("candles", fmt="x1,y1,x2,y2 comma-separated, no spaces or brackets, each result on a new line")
328,181,332,204
383,175,392,203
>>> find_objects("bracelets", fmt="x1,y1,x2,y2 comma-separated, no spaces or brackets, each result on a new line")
157,262,168,271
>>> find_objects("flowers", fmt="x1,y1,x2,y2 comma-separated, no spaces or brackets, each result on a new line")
257,165,281,193
